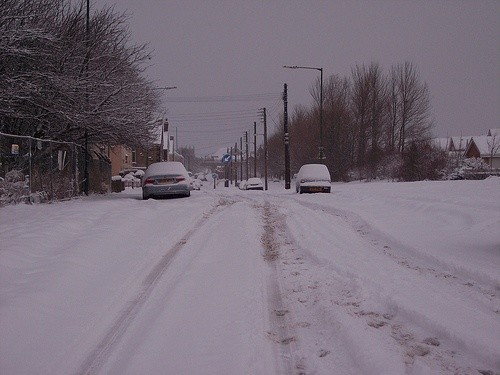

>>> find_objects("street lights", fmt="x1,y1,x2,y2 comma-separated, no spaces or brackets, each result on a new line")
282,65,324,163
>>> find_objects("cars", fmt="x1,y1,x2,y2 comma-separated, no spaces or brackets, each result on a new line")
141,161,192,201
246,178,263,191
191,171,207,192
238,181,246,190
293,164,331,195
